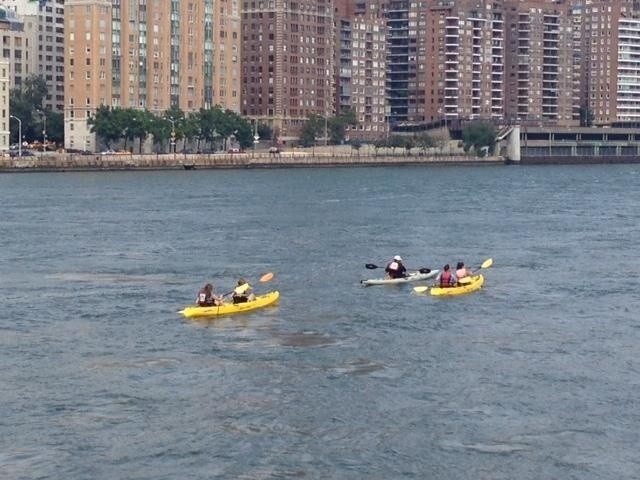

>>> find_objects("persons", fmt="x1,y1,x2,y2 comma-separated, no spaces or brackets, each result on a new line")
384,254,410,279
194,282,224,306
230,277,257,305
454,261,474,280
435,264,458,287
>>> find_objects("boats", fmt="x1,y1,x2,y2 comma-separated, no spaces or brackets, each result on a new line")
414,274,484,296
182,290,279,316
360,269,440,288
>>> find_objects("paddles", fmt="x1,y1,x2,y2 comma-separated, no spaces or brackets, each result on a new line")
178,283,249,314
223,272,273,297
413,277,471,292
472,258,492,273
366,264,430,274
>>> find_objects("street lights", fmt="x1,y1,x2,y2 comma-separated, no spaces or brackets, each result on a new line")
10,115,22,158
162,114,184,156
131,117,154,153
36,108,46,152
245,113,262,152
379,110,398,151
315,113,329,146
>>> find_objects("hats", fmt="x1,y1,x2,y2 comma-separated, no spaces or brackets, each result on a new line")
394,255,402,260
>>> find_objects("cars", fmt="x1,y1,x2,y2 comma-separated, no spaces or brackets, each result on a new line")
268,146,280,153
180,146,215,154
97,148,134,155
10,140,63,157
